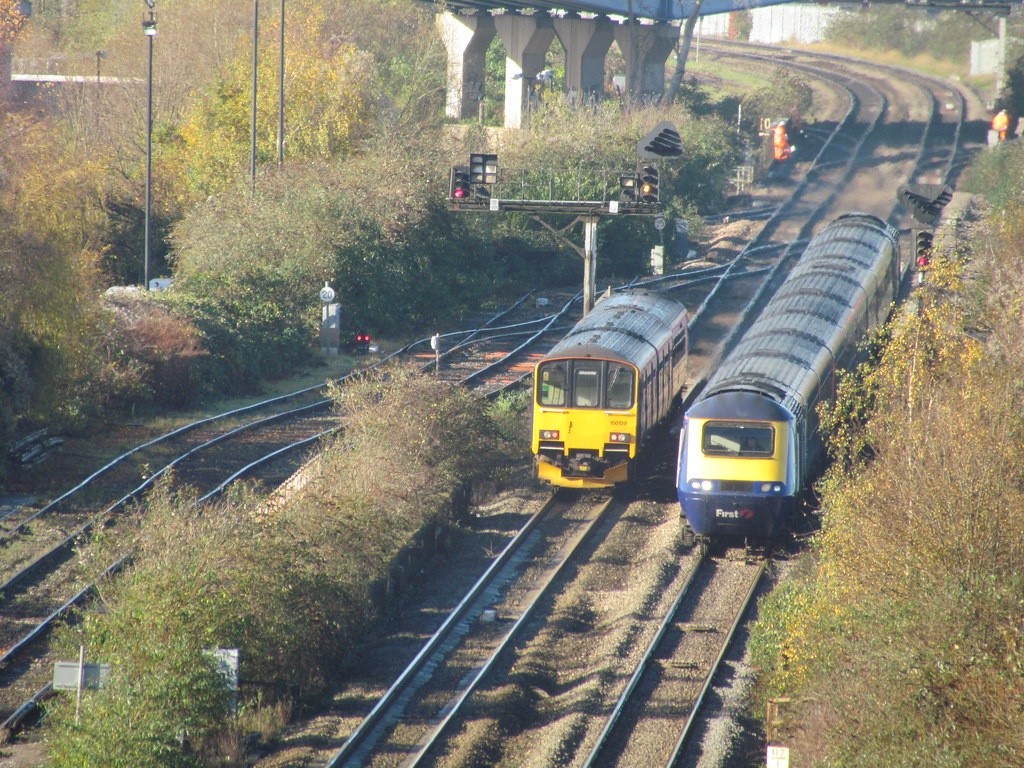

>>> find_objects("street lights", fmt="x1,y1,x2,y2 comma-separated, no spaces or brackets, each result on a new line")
141,3,160,294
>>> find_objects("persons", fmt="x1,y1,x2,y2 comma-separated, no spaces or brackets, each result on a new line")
992,109,1009,141
768,121,792,180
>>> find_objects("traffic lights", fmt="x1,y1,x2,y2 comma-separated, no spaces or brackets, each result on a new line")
913,227,937,273
449,165,471,199
619,174,638,206
637,162,660,203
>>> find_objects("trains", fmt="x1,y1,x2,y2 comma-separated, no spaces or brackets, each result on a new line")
529,285,693,492
672,211,901,538
